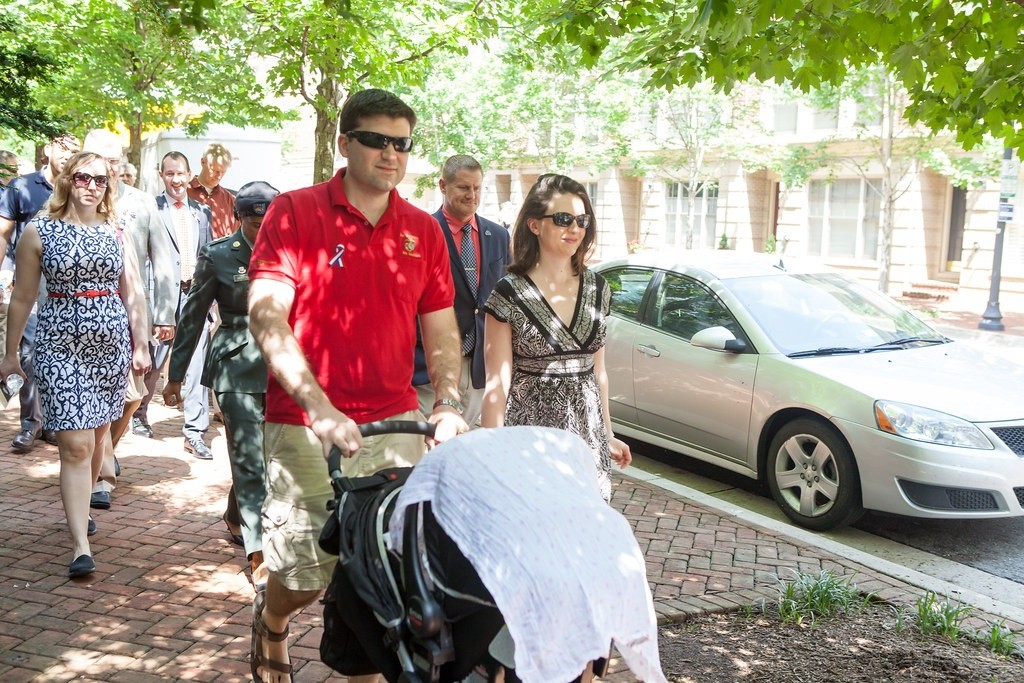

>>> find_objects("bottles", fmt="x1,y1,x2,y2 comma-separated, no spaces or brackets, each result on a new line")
6,373,24,394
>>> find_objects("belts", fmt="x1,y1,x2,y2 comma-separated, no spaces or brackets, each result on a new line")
464,349,474,357
180,280,193,290
48,290,121,298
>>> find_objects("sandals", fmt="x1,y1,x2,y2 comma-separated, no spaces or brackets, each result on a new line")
250,589,294,683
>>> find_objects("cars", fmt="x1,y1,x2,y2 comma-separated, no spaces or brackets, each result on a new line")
585,251,1024,533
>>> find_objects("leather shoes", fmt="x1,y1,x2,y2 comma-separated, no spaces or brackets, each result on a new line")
11,427,42,449
39,427,58,445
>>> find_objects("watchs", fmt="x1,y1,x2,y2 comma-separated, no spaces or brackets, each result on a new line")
433,398,464,415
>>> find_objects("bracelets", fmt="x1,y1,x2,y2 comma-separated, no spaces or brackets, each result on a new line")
607,432,614,442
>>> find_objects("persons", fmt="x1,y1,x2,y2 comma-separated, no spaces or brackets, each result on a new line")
249,89,467,683
0,129,237,508
161,182,280,592
0,151,152,579
413,155,513,430
484,174,632,510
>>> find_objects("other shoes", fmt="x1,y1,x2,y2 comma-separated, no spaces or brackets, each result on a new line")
223,510,245,547
213,410,225,424
250,566,268,593
68,550,95,577
87,513,96,535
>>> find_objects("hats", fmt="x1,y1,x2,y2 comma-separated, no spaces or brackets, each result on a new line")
84,129,122,159
235,180,282,219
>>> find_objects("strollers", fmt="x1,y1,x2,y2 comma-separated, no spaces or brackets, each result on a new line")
316,417,636,683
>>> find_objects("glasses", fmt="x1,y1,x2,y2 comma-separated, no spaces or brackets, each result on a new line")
88,154,120,165
70,172,109,188
346,129,414,152
538,212,591,228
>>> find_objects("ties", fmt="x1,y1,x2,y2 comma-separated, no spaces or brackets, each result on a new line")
461,225,478,354
174,201,192,282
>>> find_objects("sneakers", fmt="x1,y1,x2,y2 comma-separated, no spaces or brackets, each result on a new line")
114,454,120,477
90,478,111,510
132,415,153,438
184,436,212,458
